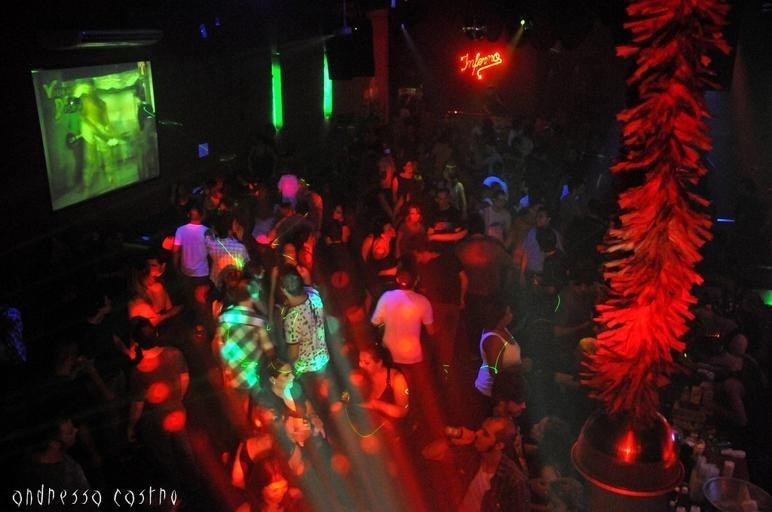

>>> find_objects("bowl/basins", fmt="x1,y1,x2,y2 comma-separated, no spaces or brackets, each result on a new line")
701,475,772,512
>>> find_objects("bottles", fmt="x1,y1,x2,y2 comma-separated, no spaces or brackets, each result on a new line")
669,484,701,512
